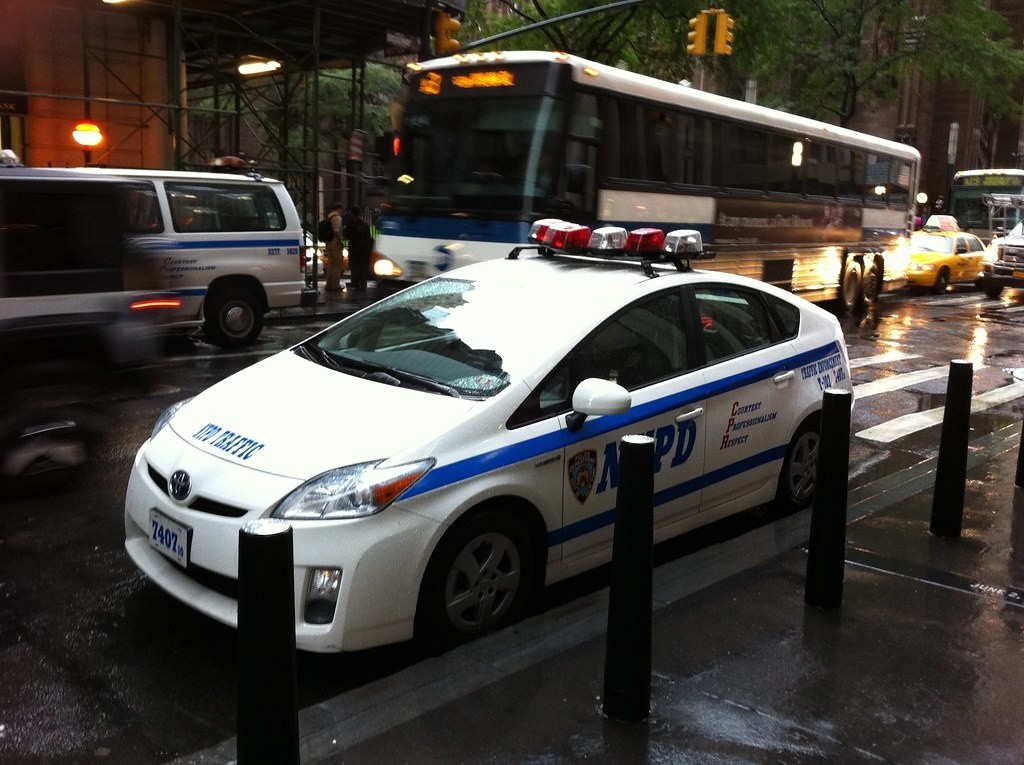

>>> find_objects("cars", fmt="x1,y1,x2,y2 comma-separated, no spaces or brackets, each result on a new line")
908,215,986,295
301,228,350,283
125,219,854,654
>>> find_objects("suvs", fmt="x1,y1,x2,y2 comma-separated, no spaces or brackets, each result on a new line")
978,218,1024,297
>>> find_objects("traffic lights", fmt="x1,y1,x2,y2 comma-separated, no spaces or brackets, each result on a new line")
687,13,707,54
433,11,461,55
713,12,734,55
375,131,400,162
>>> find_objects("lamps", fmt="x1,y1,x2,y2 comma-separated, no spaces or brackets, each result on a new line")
236,54,284,78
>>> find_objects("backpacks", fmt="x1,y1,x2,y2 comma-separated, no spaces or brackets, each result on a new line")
318,213,337,242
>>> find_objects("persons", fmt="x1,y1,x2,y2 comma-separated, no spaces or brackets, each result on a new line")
344,208,373,298
324,203,345,291
935,198,943,209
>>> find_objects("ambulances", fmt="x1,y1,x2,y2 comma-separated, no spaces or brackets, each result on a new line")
0,149,306,349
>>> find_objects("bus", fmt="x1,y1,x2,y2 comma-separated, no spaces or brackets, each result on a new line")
951,169,1024,248
369,50,921,313
